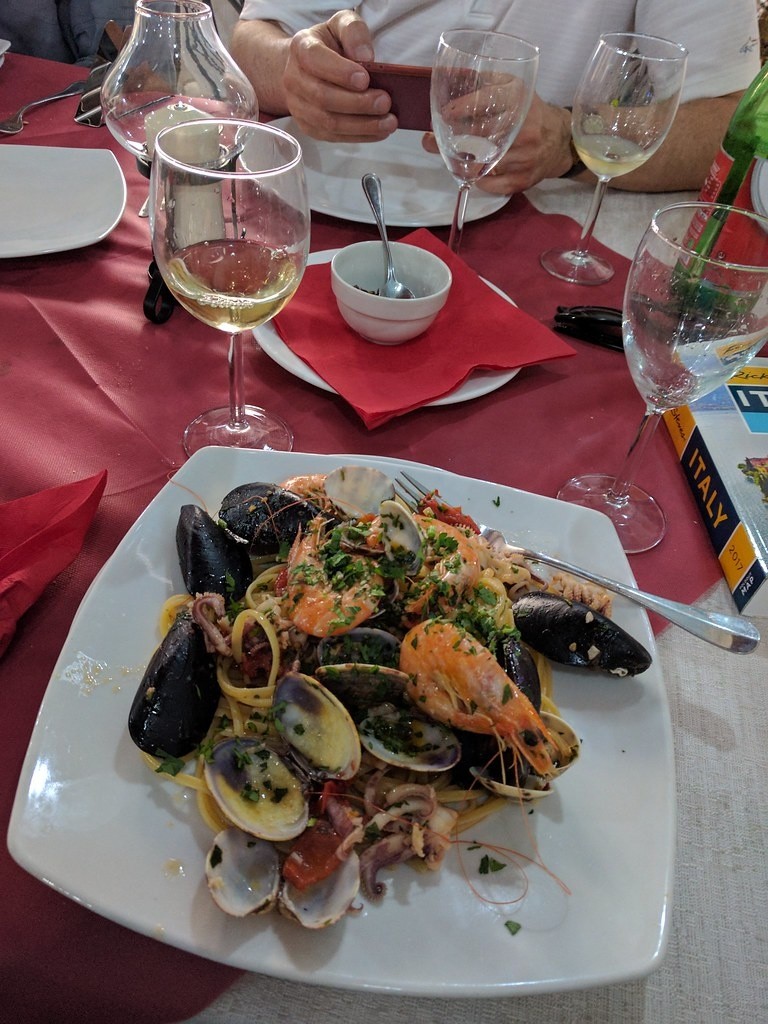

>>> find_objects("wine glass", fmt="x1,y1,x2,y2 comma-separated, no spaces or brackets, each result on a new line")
536,35,687,286
150,120,311,455
559,199,768,553
432,31,539,249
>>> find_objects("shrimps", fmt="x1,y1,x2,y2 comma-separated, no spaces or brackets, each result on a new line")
285,519,385,639
369,513,481,614
278,474,328,500
400,620,557,775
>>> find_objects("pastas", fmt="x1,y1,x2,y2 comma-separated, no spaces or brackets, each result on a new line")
140,561,561,846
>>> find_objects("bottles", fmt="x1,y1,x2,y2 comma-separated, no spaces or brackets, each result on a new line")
670,62,768,339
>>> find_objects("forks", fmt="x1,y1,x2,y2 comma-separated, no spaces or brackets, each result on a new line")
390,470,759,655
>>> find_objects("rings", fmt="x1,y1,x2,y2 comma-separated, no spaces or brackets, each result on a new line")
488,163,498,175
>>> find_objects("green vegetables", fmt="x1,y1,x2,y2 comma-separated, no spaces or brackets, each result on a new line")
148,499,604,915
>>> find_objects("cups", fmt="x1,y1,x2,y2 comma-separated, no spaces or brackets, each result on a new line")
102,0,258,164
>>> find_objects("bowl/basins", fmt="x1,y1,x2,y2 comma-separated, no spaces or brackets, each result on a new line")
332,240,451,346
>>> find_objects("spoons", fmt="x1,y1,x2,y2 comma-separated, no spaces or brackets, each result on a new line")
0,68,89,133
359,175,415,299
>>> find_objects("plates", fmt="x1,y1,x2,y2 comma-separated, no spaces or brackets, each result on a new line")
10,444,670,995
248,249,527,407
240,117,513,226
0,145,126,256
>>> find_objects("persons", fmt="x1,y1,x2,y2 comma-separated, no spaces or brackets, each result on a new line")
232,1,761,192
0,0,180,88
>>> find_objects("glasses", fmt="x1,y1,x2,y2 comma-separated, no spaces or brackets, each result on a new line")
553,304,624,354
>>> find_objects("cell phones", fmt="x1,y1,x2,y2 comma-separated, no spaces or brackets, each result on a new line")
353,61,480,133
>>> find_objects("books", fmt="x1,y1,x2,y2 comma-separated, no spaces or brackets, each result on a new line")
660,325,768,618
